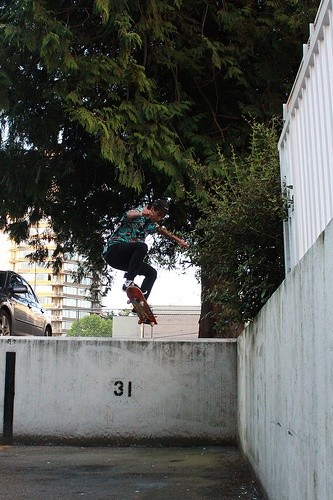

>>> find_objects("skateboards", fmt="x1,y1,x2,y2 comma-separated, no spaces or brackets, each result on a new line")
126,286,158,327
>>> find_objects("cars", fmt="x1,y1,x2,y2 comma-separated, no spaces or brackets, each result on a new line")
0,269,53,336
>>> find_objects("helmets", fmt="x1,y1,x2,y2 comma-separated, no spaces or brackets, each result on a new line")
149,198,169,214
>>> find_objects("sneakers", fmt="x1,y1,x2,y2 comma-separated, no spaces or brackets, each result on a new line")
122,279,148,295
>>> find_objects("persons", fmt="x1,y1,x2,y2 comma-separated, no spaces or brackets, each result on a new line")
104,201,188,315
